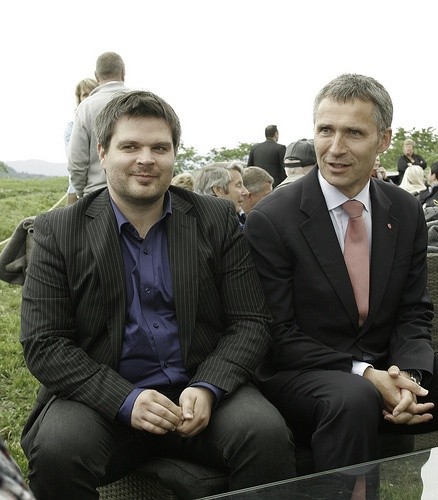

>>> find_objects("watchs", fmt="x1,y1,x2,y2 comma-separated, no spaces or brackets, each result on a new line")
402,370,421,385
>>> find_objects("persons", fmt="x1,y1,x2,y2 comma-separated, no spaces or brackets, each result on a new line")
19,91,298,500
398,139,427,184
248,125,287,189
244,73,438,500
67,53,130,198
171,161,274,226
371,155,387,181
65,78,98,206
275,139,318,190
398,162,438,210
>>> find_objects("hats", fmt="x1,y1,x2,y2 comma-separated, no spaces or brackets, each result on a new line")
283,139,316,167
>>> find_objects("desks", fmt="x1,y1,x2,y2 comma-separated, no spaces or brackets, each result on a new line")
200,448,430,500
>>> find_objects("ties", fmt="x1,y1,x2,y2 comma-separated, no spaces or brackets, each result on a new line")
342,200,370,328
